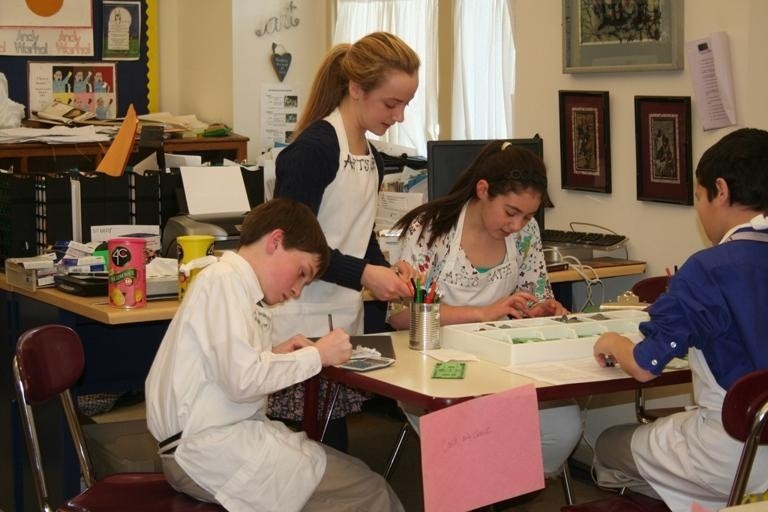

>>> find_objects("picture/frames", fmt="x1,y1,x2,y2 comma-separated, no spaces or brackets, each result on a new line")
25,59,119,121
560,0,686,75
558,89,612,194
632,94,694,206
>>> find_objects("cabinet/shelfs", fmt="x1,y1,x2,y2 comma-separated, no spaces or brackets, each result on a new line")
0,117,253,173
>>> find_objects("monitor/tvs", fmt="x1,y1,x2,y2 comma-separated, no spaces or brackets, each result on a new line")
426,138,545,233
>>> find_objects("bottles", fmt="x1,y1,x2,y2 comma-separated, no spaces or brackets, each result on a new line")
108,238,148,310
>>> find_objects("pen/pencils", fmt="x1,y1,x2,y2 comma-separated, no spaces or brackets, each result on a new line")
666,268,671,278
328,314,333,332
675,266,677,274
406,271,441,303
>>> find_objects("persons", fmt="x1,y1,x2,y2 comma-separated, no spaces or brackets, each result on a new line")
264,31,421,455
144,197,405,512
386,141,582,478
589,128,768,501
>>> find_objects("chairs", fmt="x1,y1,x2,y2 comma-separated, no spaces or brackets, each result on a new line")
12,322,225,512
563,371,767,511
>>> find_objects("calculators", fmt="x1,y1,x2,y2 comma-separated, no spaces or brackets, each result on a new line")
338,356,396,372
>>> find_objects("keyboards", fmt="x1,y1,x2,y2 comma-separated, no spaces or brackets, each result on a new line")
542,228,628,251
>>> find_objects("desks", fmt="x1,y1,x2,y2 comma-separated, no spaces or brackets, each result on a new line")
1,255,697,504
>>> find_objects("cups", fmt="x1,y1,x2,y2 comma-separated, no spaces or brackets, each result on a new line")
176,234,215,302
408,302,441,350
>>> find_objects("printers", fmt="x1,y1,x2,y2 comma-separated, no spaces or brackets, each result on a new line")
161,164,264,263
